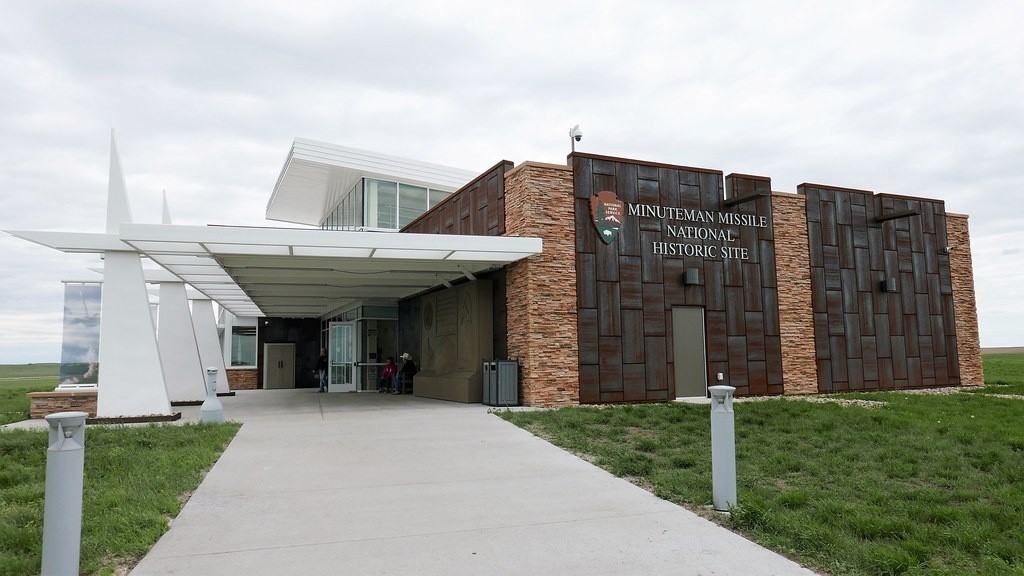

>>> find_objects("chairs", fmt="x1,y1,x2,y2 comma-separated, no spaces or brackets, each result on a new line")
391,361,419,394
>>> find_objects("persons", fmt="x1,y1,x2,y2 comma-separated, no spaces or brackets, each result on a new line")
313,347,328,393
392,353,417,394
378,356,397,394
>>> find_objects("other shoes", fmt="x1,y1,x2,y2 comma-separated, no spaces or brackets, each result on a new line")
318,390,324,393
384,389,390,394
377,389,383,393
392,391,400,395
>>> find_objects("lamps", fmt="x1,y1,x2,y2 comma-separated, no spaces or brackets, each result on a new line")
686,268,699,284
886,277,896,293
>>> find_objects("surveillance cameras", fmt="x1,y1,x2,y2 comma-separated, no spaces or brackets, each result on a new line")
573,130,583,142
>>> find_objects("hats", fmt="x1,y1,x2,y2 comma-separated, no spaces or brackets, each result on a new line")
400,353,413,360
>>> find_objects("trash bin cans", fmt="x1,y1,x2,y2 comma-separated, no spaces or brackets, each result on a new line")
482,360,519,407
